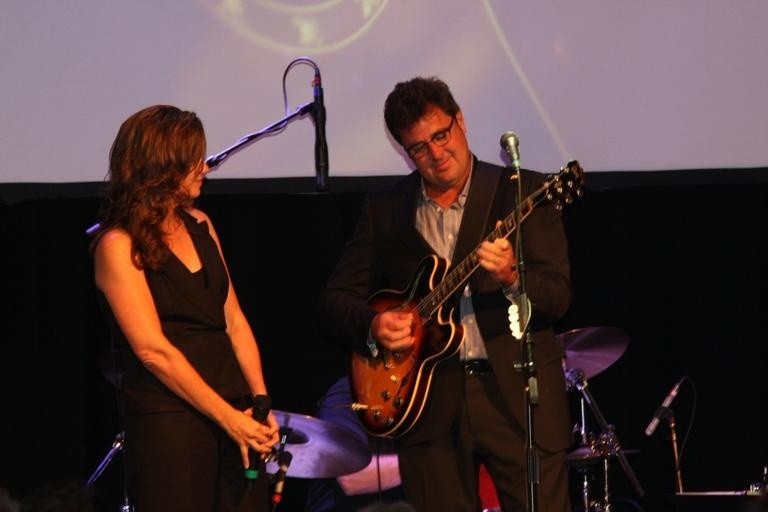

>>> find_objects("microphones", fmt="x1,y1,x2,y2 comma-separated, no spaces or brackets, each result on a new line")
245,397,271,486
644,368,686,442
501,131,524,176
306,70,336,187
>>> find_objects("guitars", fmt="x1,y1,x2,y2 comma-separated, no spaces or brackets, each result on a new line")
350,159,586,440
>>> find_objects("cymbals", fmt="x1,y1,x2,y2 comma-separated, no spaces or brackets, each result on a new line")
566,447,637,458
556,326,630,385
267,407,371,479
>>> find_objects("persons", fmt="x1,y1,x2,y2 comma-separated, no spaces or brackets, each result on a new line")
328,77,572,511
80,105,280,512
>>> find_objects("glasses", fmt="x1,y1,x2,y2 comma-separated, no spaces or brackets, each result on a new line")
408,117,456,158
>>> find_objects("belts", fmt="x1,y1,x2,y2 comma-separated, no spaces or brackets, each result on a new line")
457,358,495,375
